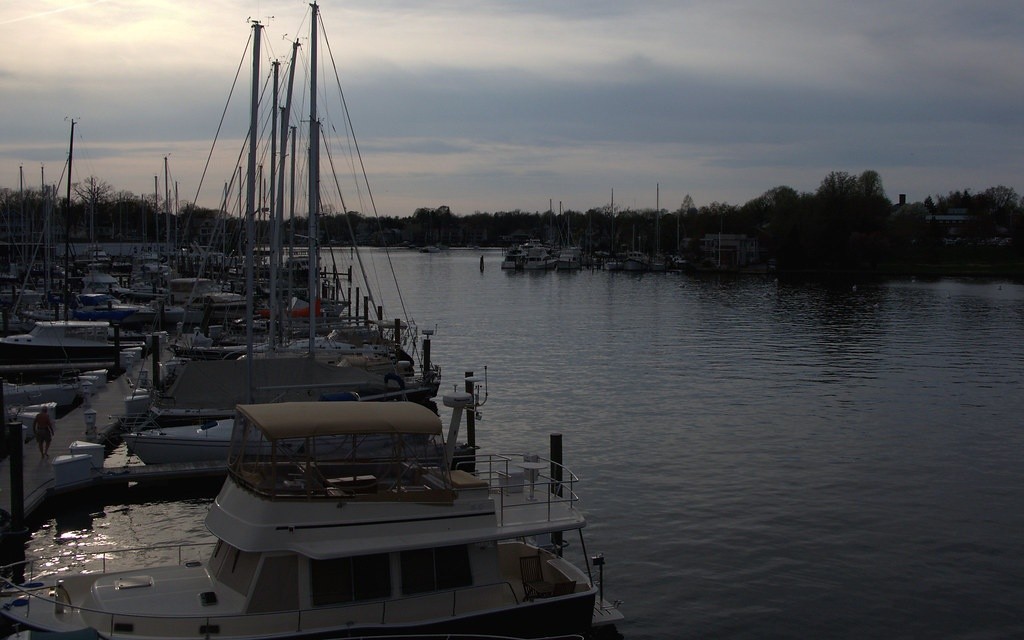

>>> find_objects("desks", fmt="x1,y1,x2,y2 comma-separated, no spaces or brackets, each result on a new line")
515,463,549,500
325,475,378,494
527,581,555,597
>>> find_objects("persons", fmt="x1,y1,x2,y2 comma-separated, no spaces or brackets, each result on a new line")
33,407,55,458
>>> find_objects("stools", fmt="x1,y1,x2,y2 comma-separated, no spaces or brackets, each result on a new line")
498,469,524,492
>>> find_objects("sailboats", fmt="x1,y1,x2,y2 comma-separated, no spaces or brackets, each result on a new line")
545,180,722,274
0,0,489,485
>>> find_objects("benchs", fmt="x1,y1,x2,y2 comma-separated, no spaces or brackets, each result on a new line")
437,470,490,499
306,462,421,490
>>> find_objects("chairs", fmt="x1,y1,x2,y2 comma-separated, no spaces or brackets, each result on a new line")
554,581,576,597
518,554,544,602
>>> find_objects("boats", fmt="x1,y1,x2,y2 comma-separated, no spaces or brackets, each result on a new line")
419,246,440,254
501,248,528,268
409,245,416,250
438,244,449,250
0,383,624,640
523,247,557,269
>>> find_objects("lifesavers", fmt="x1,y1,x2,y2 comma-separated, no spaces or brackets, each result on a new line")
346,448,383,486
